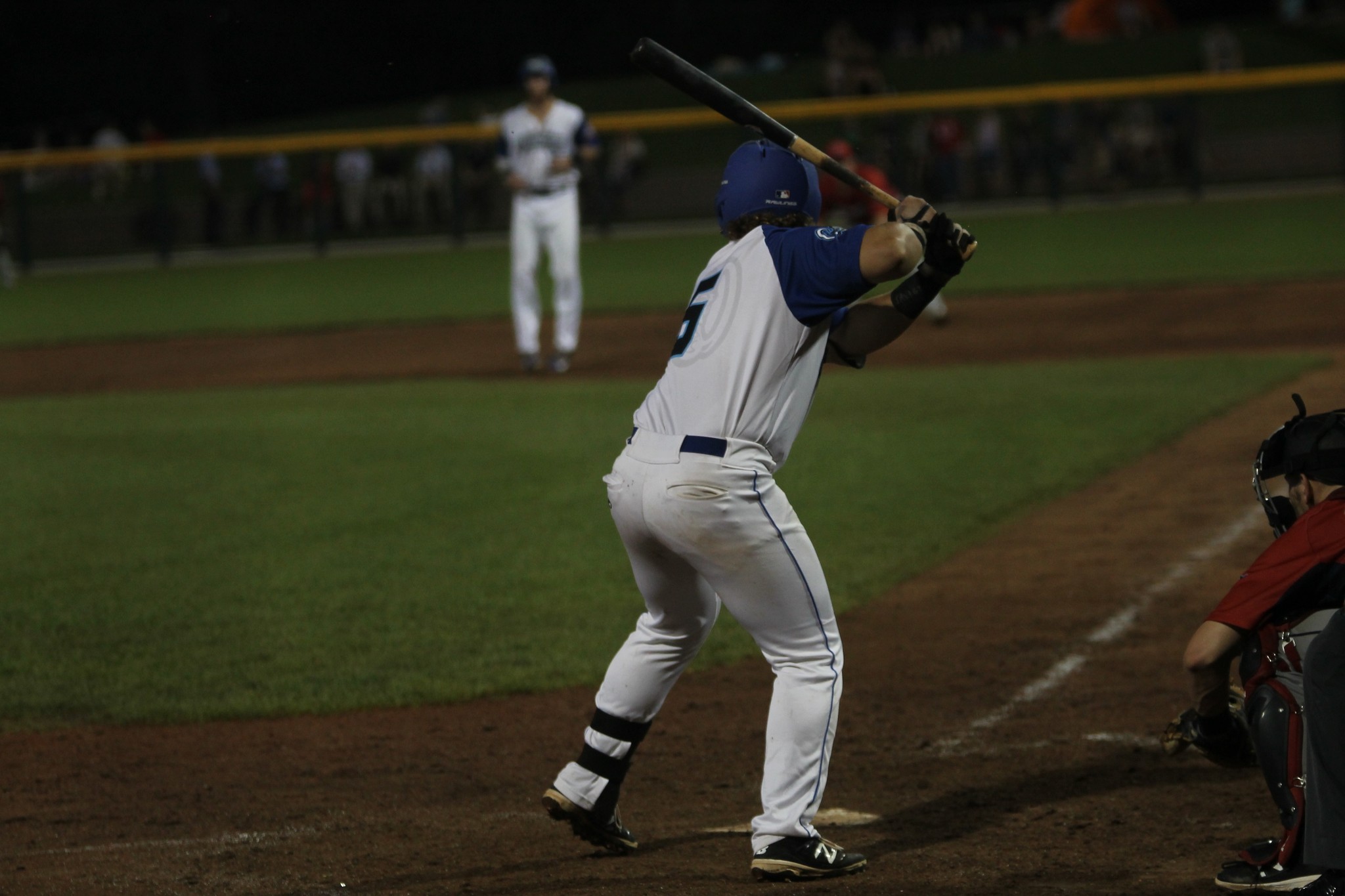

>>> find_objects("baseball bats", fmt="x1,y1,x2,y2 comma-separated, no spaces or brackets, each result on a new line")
629,35,979,260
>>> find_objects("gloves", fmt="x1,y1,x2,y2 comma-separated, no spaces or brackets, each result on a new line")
887,194,978,291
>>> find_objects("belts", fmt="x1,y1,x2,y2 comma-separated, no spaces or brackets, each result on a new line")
627,427,728,456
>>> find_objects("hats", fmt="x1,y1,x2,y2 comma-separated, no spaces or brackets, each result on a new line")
527,57,556,79
825,141,853,160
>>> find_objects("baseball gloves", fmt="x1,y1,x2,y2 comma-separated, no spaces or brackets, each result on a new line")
1189,683,1257,767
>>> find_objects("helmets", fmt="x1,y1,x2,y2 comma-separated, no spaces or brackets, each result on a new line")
1260,408,1345,485
711,138,823,240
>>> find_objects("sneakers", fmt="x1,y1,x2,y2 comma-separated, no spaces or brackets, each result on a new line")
1214,861,1323,892
751,835,868,882
541,784,640,852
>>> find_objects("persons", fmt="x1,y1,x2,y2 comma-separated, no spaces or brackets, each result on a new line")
1182,393,1345,896
89,0,1211,248
544,134,976,875
496,61,597,375
816,138,953,322
